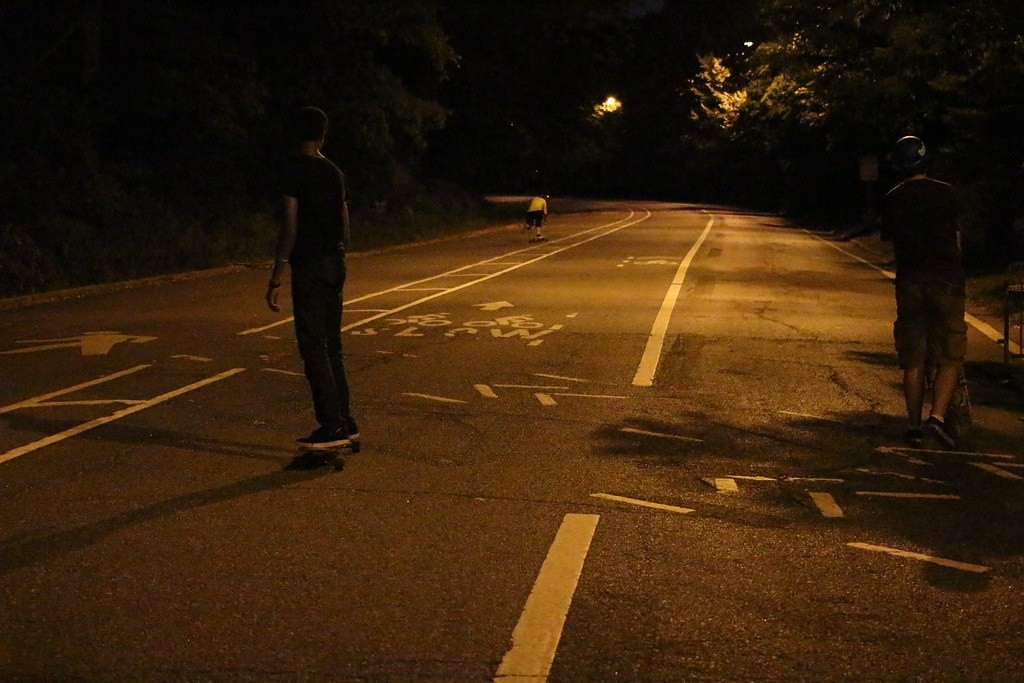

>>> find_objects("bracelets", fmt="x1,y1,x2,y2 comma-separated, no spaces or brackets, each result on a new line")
272,284,283,288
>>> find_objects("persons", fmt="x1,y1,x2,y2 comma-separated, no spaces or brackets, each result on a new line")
880,137,966,448
522,192,547,242
266,107,361,453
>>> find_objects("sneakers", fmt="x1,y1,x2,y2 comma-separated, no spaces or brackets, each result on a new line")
906,426,923,443
296,421,359,447
926,416,956,449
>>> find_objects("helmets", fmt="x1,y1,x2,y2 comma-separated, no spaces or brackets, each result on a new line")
892,135,930,176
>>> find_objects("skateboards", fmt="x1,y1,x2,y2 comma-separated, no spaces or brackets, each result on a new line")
529,238,548,244
926,334,972,439
293,437,362,471
